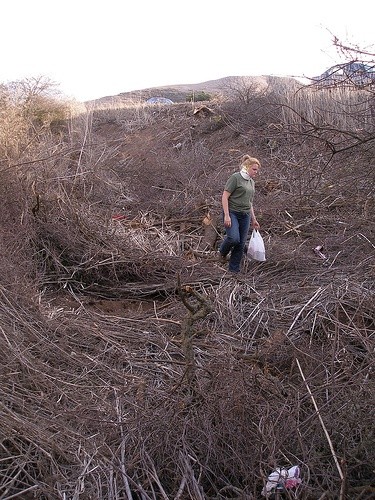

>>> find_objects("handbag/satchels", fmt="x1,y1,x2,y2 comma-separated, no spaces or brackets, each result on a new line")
247,228,267,262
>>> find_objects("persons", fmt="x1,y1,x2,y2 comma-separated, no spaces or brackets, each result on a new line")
217,153,261,273
